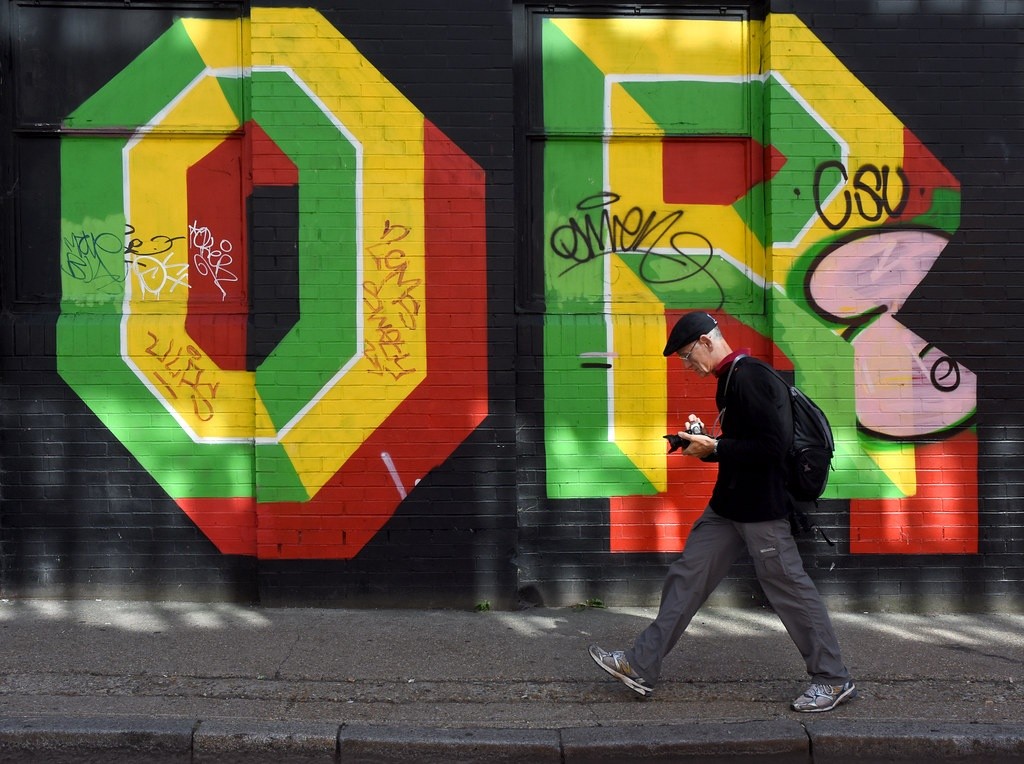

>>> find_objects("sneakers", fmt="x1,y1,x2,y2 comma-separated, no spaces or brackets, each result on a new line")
790,681,858,713
587,644,655,698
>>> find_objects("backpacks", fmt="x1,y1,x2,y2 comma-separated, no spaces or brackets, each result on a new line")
731,357,836,505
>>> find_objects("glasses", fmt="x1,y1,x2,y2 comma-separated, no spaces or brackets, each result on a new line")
678,336,712,362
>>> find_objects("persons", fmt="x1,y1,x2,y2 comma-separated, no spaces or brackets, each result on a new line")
588,310,856,712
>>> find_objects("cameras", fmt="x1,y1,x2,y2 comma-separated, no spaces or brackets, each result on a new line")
662,421,704,454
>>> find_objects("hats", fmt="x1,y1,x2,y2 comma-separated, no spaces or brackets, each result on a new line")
663,310,718,357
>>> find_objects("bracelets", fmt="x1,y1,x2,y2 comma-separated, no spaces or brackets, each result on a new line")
712,440,718,454
